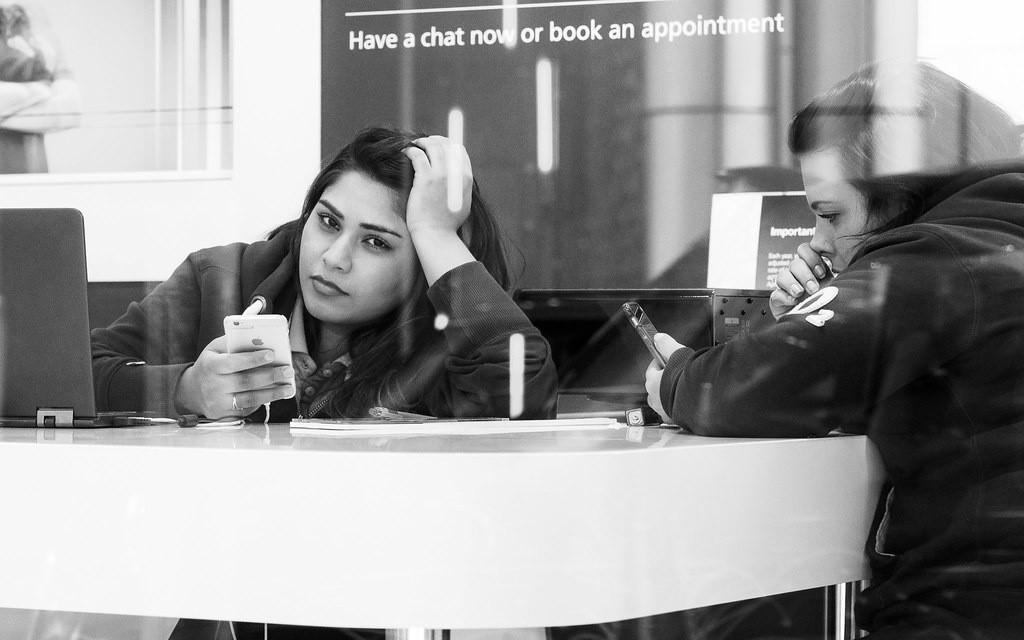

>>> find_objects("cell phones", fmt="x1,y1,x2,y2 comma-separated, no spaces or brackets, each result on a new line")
225,315,297,400
623,302,667,370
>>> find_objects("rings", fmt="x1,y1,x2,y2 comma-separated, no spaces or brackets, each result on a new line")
232,394,243,412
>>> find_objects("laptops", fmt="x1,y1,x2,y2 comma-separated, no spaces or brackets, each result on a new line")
0,208,159,427
509,286,716,420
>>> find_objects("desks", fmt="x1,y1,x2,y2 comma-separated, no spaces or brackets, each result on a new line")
0,422,888,640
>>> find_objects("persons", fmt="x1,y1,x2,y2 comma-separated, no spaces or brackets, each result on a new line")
89,125,559,422
642,55,1024,640
